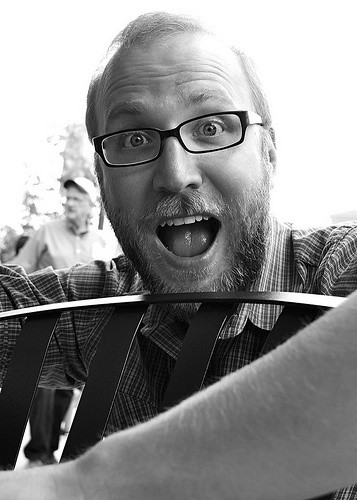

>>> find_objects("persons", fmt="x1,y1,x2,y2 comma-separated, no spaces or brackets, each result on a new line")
1,14,356,500
2,175,120,467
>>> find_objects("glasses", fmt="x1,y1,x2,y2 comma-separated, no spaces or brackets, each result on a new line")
92,110,264,167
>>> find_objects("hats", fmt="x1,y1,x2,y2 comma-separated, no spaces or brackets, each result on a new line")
64,176,97,202
